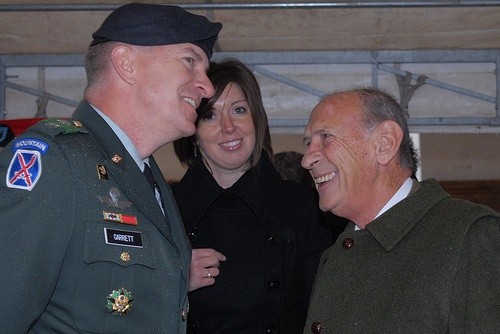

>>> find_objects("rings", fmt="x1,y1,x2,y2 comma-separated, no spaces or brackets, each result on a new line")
207,268,211,278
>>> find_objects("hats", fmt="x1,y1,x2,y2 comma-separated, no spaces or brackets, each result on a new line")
91,3,222,60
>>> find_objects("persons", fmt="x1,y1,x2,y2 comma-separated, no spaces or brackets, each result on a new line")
165,56,349,334
274,150,317,192
302,88,500,334
0,2,223,334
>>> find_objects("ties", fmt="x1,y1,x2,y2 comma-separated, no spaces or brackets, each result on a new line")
143,163,165,218
